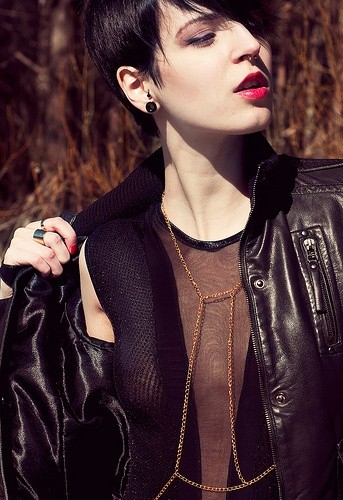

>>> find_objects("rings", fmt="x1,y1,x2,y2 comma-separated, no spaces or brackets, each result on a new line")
41,217,48,231
33,229,46,245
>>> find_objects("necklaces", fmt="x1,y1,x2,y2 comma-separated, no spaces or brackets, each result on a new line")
151,186,275,499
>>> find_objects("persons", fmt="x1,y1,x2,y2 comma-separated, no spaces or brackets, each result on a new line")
0,0,343,500
